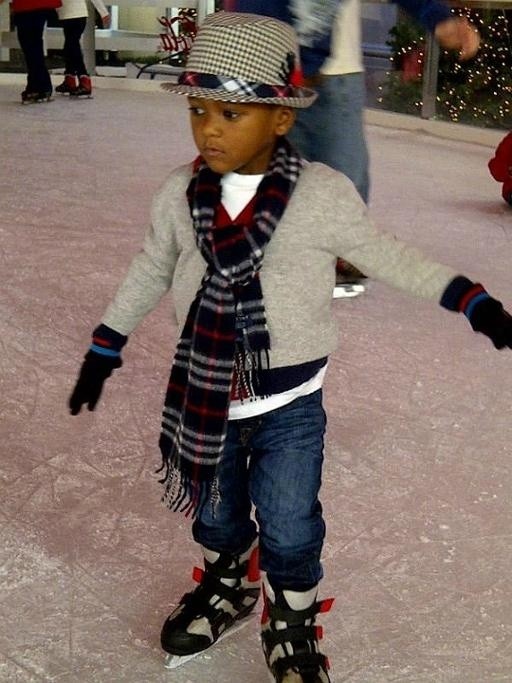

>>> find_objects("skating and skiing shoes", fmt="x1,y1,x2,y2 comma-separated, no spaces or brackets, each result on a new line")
21,73,52,105
330,256,370,300
55,71,94,99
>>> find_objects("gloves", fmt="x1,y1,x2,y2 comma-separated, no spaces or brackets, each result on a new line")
439,272,512,353
63,323,128,418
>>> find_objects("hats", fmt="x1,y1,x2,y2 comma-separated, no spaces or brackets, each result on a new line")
157,10,321,110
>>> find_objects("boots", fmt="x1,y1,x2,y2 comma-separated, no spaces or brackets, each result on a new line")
159,516,264,671
258,558,333,683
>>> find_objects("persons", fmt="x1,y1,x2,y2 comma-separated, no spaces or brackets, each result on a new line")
217,0,483,300
54,0,112,96
8,0,64,100
65,11,512,682
